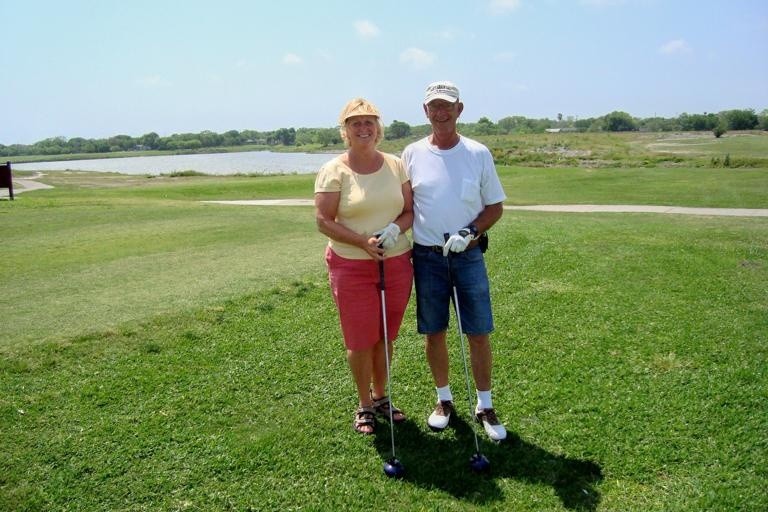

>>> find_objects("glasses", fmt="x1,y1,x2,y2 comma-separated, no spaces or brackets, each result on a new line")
427,102,456,111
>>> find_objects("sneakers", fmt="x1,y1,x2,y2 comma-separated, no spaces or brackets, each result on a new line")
474,406,507,441
427,400,453,429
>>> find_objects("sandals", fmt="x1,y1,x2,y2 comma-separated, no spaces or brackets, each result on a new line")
354,406,376,433
369,389,406,423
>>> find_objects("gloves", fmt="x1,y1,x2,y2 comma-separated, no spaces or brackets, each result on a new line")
443,228,475,257
373,222,401,250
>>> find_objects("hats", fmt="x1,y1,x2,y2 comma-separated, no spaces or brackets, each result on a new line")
344,99,379,122
423,82,460,104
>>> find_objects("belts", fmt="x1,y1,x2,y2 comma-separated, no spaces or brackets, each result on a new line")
428,240,478,252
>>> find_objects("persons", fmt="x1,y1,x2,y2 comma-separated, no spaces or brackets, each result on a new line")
400,80,510,442
312,97,415,436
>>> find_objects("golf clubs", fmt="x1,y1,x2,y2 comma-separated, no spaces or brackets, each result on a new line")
375,234,402,476
444,233,490,469
